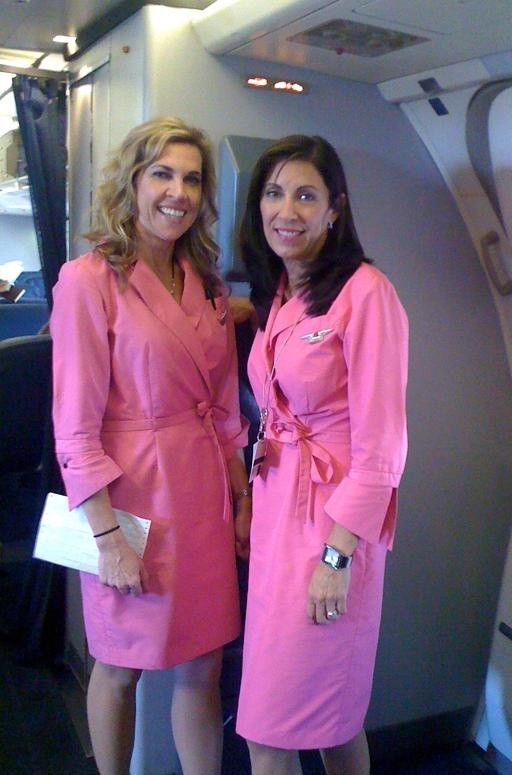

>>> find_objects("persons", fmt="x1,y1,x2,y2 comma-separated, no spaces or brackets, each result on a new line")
48,116,256,774
233,135,411,774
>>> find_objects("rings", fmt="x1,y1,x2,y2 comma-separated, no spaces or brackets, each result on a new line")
327,609,335,616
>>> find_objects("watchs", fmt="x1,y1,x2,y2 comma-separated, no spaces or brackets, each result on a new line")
319,543,354,571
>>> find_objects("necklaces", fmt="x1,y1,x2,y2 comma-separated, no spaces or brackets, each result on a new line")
165,258,181,299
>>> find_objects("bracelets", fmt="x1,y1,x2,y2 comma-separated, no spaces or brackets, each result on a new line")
231,487,253,501
93,524,123,538
